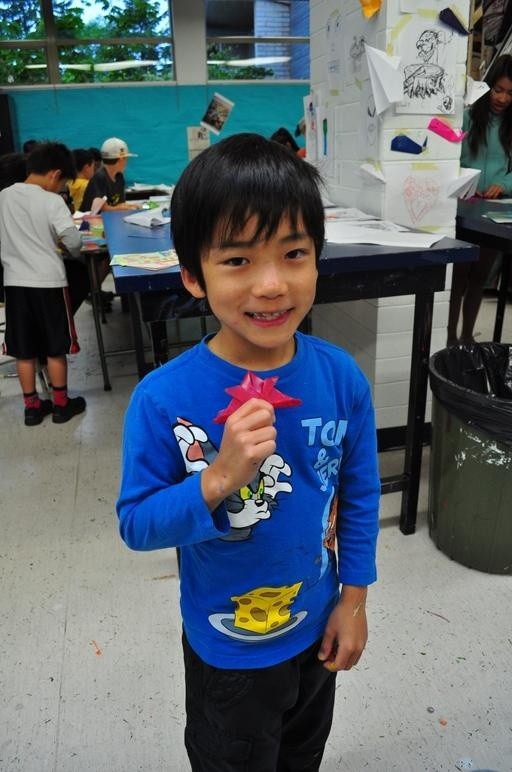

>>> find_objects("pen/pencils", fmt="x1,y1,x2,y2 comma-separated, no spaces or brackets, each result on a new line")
128,236,157,239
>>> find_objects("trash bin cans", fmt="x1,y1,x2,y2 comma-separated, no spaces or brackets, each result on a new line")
427,342,512,574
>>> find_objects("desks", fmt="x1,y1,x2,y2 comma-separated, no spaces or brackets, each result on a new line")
97,208,485,539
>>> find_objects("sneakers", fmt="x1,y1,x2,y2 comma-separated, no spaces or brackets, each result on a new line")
24,399,51,427
84,288,114,314
52,397,86,423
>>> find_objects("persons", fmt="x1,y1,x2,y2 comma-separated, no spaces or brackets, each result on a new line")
1,138,88,426
445,54,512,350
270,128,306,159
1,140,102,216
113,134,382,771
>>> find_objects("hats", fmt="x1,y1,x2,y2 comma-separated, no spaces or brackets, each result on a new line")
101,137,138,160
